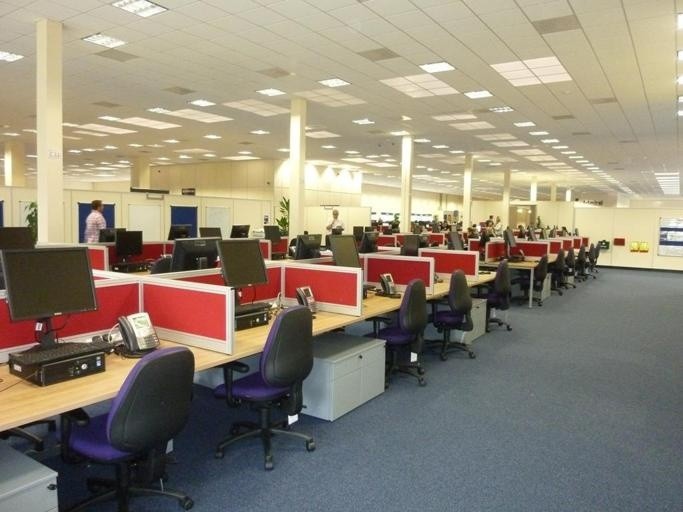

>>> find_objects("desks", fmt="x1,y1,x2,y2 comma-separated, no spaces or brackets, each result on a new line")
434,279,478,299
479,271,497,285
488,259,539,309
231,310,360,363
360,288,434,320
0,338,231,432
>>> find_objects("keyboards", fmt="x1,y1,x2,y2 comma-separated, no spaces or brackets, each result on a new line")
7,339,113,365
235,301,273,315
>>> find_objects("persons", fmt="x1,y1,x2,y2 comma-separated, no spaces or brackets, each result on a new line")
327,209,345,235
83,199,106,243
468,215,503,238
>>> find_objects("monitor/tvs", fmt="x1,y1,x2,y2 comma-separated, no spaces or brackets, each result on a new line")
353,218,580,257
1,225,34,249
0,246,100,350
95,222,363,271
215,238,268,307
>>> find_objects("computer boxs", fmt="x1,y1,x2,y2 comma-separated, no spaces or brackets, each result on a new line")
9,352,106,387
234,310,268,331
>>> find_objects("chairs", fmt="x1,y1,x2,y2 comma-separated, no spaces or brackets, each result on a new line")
375,278,428,388
212,306,315,471
67,346,195,512
473,259,513,333
428,269,476,361
511,242,602,306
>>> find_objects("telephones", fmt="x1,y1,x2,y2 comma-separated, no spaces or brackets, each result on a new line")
380,272,398,295
118,311,160,353
296,286,318,314
